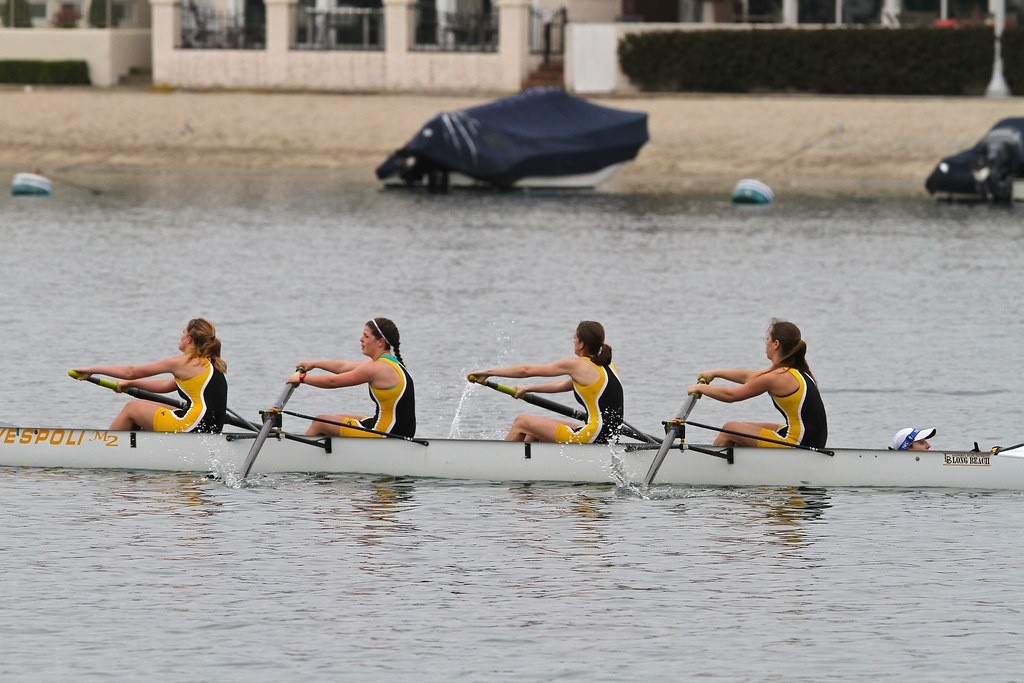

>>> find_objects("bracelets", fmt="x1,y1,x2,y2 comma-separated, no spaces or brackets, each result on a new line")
300,374,306,382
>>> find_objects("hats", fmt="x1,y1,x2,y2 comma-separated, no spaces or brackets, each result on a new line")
887,427,936,451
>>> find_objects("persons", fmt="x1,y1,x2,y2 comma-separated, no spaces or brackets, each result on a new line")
894,427,936,449
285,318,416,437
467,321,624,443
75,319,228,434
687,322,828,448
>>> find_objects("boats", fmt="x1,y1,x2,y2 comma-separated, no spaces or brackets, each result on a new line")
924,118,1023,202
373,83,649,190
0,426,1024,491
